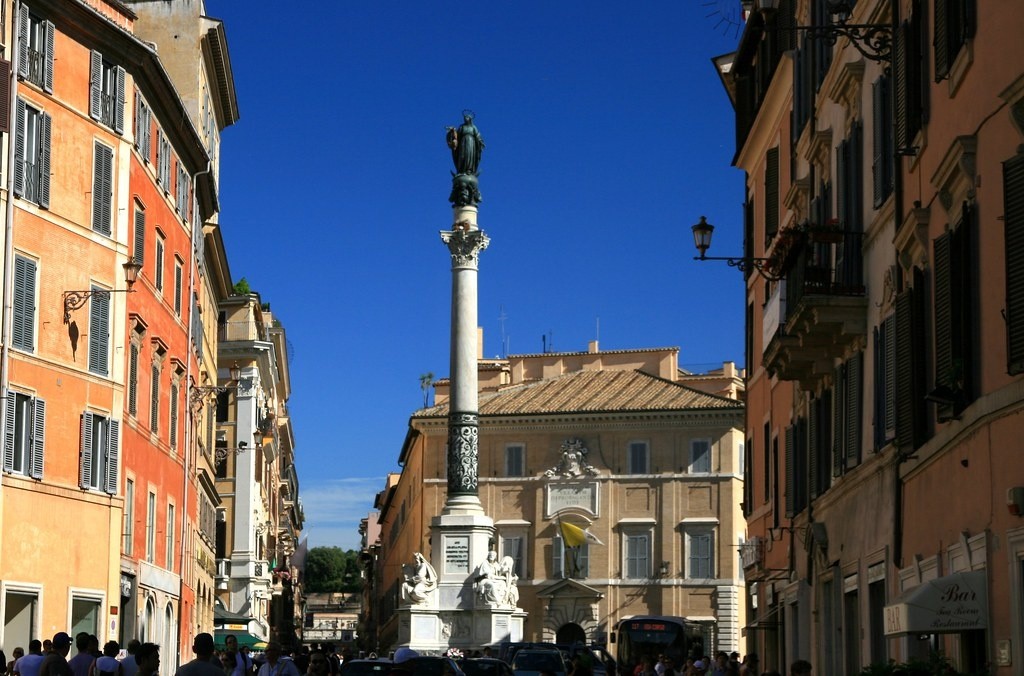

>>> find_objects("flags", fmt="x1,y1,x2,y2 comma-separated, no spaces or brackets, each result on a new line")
560,520,606,548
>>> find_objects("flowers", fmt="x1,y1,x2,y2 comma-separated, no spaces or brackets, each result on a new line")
766,218,840,268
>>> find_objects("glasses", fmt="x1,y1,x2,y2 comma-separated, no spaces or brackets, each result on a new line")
264,649,271,652
227,640,236,643
70,643,72,645
221,659,227,662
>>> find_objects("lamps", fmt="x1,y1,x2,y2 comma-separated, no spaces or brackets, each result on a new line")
692,216,791,283
658,561,670,575
64,256,144,323
214,426,264,467
756,568,790,579
189,361,243,408
924,384,963,408
756,0,894,64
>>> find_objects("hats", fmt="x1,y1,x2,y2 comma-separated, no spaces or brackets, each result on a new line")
53,632,73,643
693,661,703,667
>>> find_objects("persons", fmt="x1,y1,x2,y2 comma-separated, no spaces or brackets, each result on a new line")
174,634,813,676
0,632,160,676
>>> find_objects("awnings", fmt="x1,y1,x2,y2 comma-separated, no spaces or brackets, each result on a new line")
883,568,987,638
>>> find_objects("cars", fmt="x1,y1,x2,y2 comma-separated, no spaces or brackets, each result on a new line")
511,648,567,676
339,649,466,676
456,657,514,676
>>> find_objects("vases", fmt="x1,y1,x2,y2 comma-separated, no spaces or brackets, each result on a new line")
807,232,842,244
777,235,802,271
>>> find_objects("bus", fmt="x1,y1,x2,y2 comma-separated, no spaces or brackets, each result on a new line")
610,616,691,676
